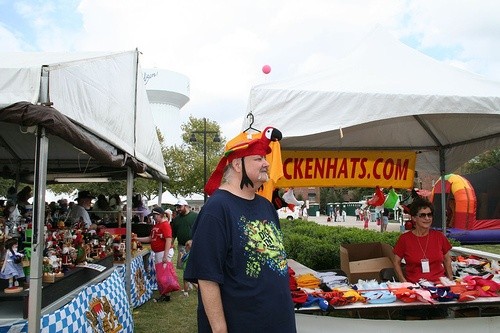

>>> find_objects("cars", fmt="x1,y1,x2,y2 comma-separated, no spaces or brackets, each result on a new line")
276,205,302,220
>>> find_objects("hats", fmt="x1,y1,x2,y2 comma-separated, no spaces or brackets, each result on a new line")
74,190,96,201
174,199,188,206
147,209,163,216
205,126,285,202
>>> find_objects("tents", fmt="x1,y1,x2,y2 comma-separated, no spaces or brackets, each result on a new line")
0,46,169,331
242,30,500,239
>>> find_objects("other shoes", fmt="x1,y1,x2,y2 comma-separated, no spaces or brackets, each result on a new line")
181,288,190,292
183,292,188,296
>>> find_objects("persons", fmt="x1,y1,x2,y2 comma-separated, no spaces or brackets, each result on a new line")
363,214,370,229
183,127,297,333
136,194,151,222
170,199,199,292
392,198,453,283
74,191,105,229
355,207,370,221
1,237,24,292
91,194,108,220
132,205,174,303
382,214,388,231
334,210,346,222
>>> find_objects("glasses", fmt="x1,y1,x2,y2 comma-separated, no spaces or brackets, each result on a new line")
413,213,433,218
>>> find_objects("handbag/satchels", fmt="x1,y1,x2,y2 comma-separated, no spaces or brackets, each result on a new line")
155,261,181,295
152,295,171,303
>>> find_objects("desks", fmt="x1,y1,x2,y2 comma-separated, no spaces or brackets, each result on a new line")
0,267,135,333
114,243,159,308
294,290,500,333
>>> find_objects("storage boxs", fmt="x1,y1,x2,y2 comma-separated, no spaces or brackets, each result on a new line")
340,242,394,284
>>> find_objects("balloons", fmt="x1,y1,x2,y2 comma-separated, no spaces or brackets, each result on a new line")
262,65,271,74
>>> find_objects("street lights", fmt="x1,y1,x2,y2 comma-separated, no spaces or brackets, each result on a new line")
189,118,221,205
